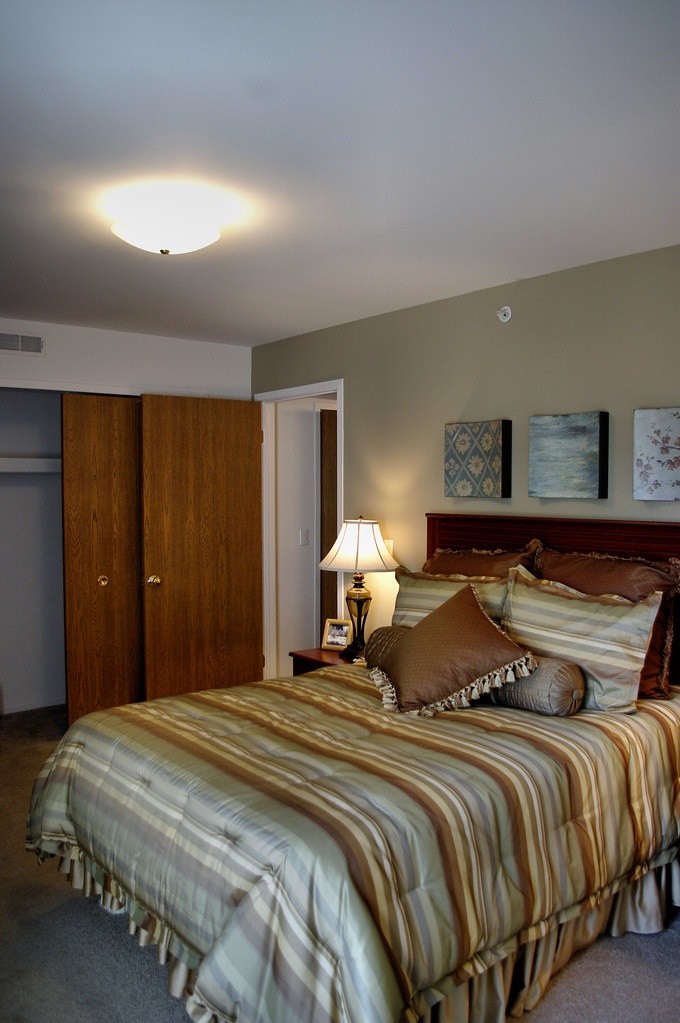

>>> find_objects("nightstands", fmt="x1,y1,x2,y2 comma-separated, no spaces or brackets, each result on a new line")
288,648,346,677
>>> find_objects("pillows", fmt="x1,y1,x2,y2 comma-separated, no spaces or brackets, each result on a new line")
534,548,680,700
500,563,663,715
392,565,507,629
421,538,540,579
366,582,538,716
363,626,586,717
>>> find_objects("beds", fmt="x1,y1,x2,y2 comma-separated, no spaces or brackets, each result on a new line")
21,512,680,1023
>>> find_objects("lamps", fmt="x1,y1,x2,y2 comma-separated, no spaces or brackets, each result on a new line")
110,214,221,257
318,516,401,663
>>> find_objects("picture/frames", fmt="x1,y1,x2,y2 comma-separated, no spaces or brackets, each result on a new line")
321,618,353,651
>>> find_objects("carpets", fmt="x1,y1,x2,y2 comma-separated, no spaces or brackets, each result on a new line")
0,894,680,1023
